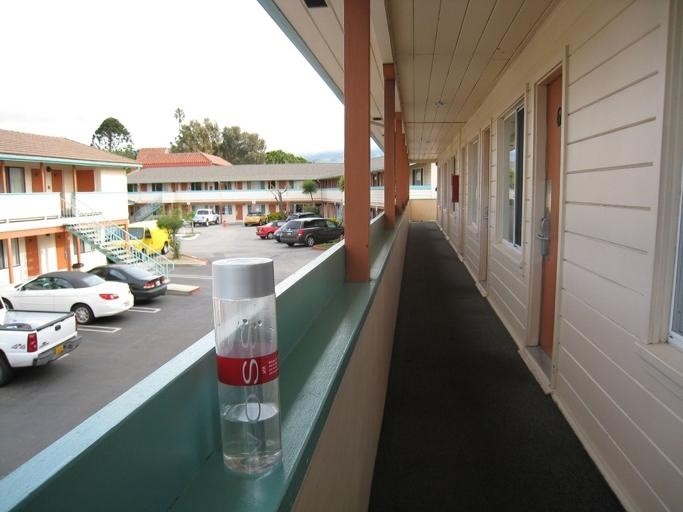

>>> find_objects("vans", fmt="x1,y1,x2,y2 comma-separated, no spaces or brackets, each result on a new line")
108,219,171,263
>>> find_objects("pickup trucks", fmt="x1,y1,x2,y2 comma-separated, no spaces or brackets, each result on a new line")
191,209,220,227
0,295,81,390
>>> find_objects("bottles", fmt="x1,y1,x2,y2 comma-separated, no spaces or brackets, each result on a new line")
210,256,284,479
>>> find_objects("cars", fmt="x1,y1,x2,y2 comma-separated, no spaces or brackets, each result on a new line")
85,264,167,301
244,211,345,248
1,271,134,324
